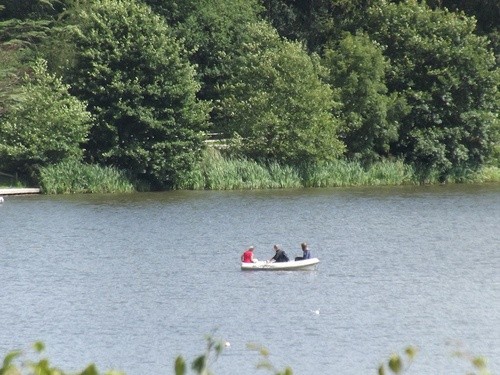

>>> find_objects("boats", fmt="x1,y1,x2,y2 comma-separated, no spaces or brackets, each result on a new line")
242,258,320,271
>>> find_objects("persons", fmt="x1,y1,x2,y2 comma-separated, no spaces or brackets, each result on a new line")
295,243,311,261
270,245,290,262
241,246,258,263
